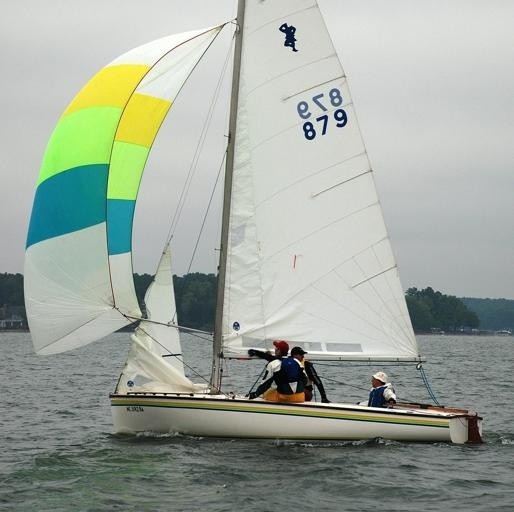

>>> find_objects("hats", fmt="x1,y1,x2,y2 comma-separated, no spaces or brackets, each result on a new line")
273,341,289,352
291,347,307,355
372,372,387,383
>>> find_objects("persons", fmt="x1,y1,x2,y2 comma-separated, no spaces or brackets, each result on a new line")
249,339,307,404
368,372,396,407
248,347,331,403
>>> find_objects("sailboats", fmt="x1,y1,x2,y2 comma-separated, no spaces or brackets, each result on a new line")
23,0,486,446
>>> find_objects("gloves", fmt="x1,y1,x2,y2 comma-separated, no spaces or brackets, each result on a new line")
248,350,255,356
245,393,256,399
322,398,329,403
